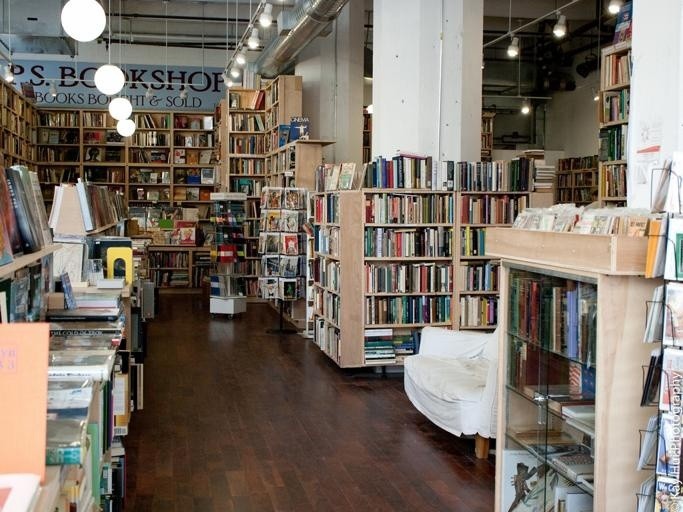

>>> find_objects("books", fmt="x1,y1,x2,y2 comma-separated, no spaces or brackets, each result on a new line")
363,113,372,163
637,212,682,510
482,4,653,236
297,149,557,363
501,269,598,511
2,86,154,512
146,80,309,301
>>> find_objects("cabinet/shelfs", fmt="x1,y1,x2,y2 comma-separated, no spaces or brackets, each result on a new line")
363,110,494,166
312,155,599,370
597,38,632,209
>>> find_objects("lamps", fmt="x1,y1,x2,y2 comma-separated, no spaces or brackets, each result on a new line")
220,0,274,88
61,0,136,139
4,0,16,84
37,1,207,101
483,17,553,117
482,0,627,72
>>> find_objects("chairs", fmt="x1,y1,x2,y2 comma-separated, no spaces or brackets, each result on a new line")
404,325,498,460
